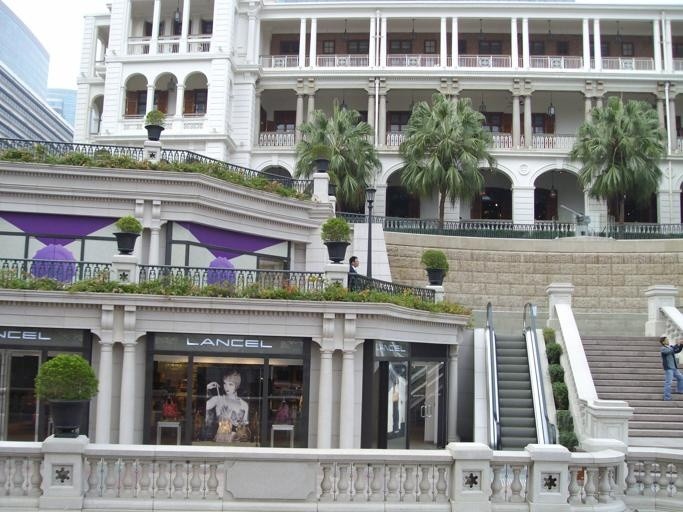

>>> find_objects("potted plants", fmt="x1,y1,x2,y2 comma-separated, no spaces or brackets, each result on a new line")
420,249,449,285
33,352,100,430
310,143,331,172
320,216,351,261
144,110,165,141
112,214,143,253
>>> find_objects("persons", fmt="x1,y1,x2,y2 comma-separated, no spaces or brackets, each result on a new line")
348,256,360,273
206,369,249,427
659,336,683,401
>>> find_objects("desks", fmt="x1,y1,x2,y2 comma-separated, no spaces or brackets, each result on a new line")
270,423,294,448
157,419,182,446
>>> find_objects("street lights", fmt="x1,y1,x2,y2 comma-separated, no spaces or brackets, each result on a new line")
366,177,377,280
